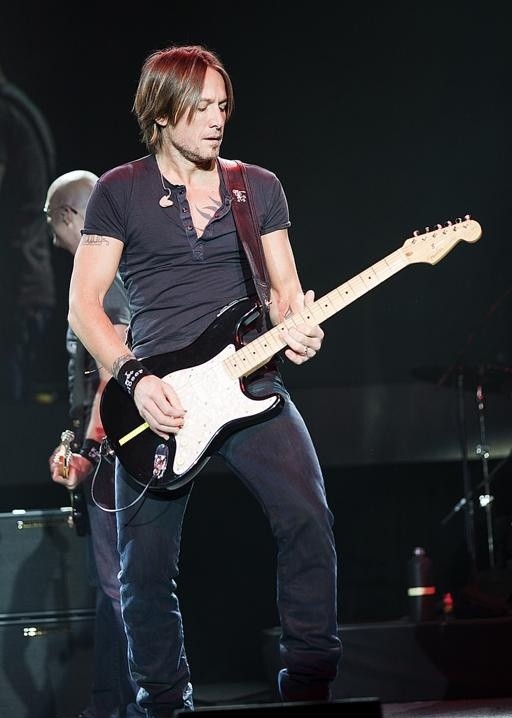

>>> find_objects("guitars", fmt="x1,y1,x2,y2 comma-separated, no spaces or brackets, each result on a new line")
99,214,482,494
59,430,91,534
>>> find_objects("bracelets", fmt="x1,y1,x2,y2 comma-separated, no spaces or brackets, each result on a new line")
79,439,103,470
117,359,153,396
113,353,137,380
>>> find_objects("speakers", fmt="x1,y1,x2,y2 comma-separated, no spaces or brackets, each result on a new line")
174,698,383,718
0,509,96,618
0,615,96,718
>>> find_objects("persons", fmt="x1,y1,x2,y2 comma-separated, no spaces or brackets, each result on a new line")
64,46,337,717
45,169,150,717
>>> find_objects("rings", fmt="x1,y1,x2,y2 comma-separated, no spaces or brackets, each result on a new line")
301,347,309,356
